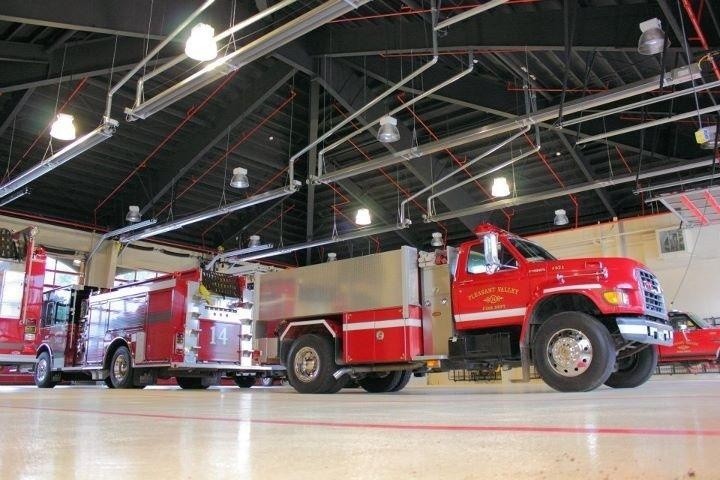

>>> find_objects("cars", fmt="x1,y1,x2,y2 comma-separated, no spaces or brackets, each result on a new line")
656,310,720,368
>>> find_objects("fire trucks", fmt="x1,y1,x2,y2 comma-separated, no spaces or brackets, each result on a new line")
33,265,258,390
251,223,677,394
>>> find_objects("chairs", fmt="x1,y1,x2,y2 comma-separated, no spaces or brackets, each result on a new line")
500,240,516,270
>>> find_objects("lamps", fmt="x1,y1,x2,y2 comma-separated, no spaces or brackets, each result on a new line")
49,74,76,142
431,231,445,248
637,18,670,55
124,204,143,223
354,207,372,226
492,176,511,197
248,234,261,247
553,208,570,226
377,26,401,144
185,11,217,61
229,165,251,189
